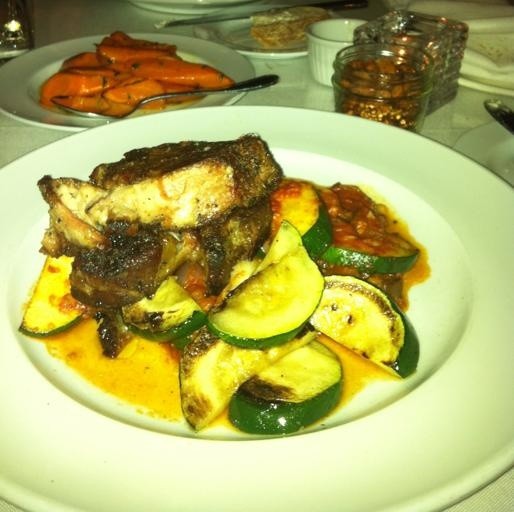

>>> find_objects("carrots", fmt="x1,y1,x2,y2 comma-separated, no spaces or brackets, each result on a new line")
41,31,235,118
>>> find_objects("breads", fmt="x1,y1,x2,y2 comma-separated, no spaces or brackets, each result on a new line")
250,6,332,49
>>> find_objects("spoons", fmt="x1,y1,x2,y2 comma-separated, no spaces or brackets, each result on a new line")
49,74,280,121
482,98,513,135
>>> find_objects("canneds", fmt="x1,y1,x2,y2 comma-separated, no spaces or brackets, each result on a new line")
331,43,435,133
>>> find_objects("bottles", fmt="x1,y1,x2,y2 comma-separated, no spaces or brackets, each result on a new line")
0,1,33,60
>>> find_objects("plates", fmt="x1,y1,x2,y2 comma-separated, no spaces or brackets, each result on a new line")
0,103,514,512
406,0,512,22
205,5,342,58
455,16,513,96
132,2,234,21
0,32,257,133
302,15,368,86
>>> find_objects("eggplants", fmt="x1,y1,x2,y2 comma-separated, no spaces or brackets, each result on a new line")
19,177,422,435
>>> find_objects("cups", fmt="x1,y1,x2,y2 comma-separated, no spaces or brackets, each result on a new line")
332,11,468,133
